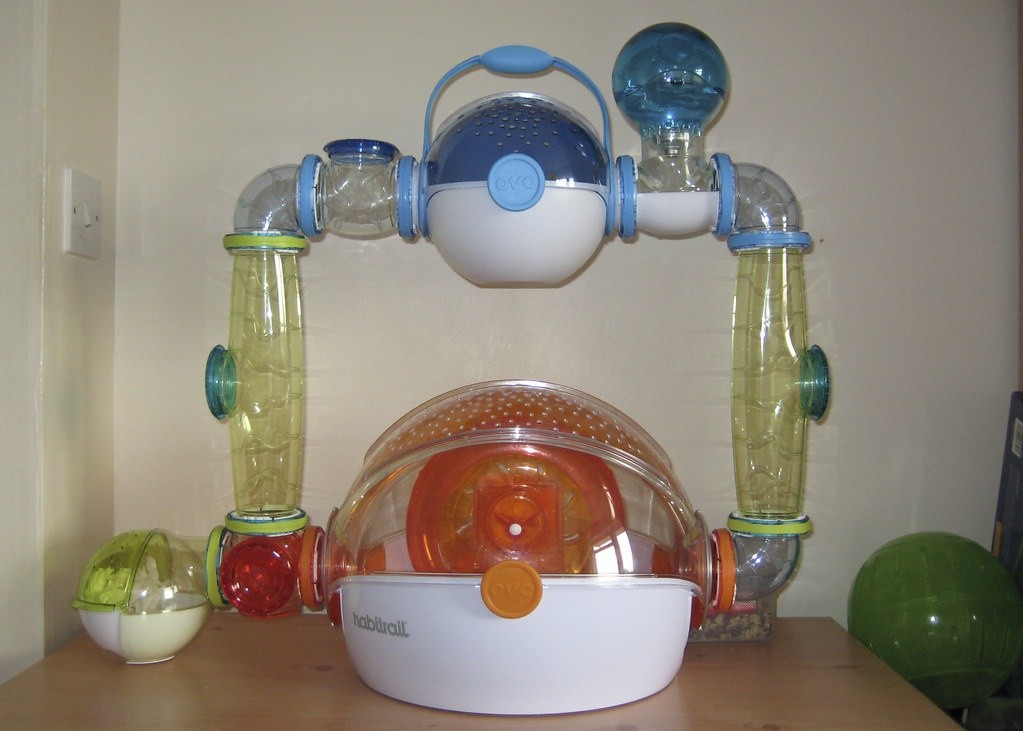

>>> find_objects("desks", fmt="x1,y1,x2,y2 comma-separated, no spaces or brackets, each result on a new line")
0,613,957,731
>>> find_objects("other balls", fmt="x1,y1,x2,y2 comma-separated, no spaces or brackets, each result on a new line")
847,531,1023,711
